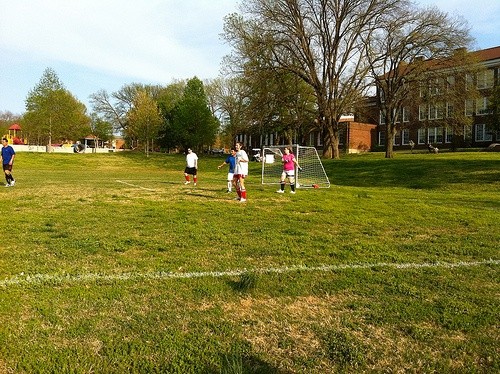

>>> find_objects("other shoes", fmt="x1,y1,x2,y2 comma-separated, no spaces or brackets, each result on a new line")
5,183,10,187
194,183,196,186
276,189,284,194
240,198,246,202
290,191,295,194
226,190,231,193
235,197,240,200
11,180,15,186
184,181,190,185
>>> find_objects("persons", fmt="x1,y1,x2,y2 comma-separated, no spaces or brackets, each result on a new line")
276,147,302,194
218,147,237,193
184,148,199,186
409,139,414,152
233,142,250,201
1,138,16,188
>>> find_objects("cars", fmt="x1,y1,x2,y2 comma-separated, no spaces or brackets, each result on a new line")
210,148,225,155
